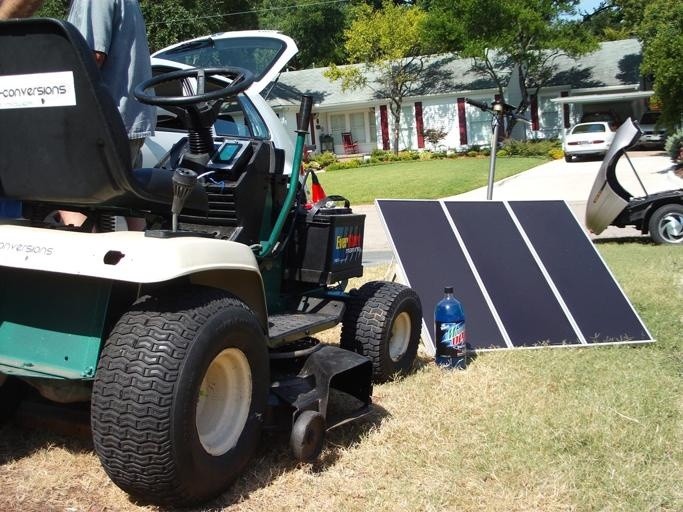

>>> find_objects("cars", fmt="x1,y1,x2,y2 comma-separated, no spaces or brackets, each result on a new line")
561,108,682,244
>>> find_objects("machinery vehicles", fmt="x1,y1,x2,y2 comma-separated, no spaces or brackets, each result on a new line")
0,14,421,508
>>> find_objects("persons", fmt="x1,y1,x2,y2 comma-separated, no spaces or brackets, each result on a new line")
0,1,46,220
66,1,158,232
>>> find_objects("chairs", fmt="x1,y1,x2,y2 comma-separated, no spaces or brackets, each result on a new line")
0,17,206,232
340,132,359,154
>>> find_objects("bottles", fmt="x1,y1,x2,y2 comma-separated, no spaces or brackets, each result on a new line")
434,284,468,371
